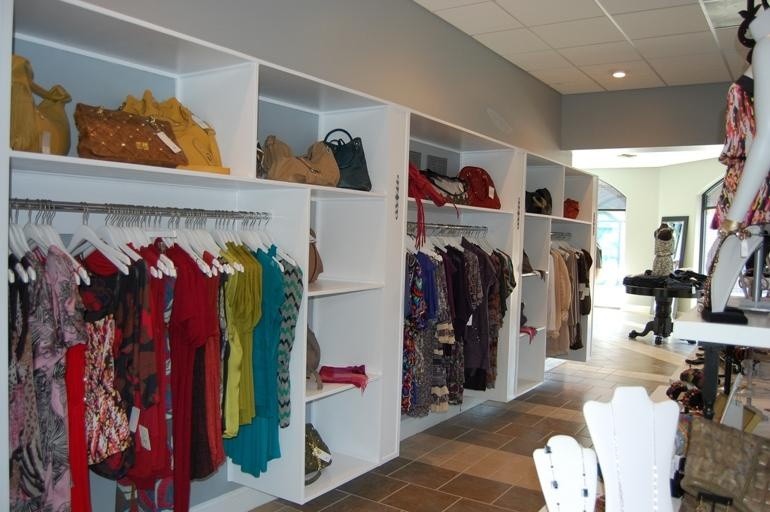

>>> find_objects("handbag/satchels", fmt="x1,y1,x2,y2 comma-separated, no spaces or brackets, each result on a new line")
256,129,372,190
11,55,231,173
308,229,324,284
522,251,534,274
305,423,333,486
306,322,323,391
409,161,502,252
676,415,770,512
525,188,552,215
563,198,579,218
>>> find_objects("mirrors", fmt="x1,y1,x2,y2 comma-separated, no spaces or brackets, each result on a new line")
650,216,689,320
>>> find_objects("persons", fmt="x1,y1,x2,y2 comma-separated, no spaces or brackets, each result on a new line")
706,1,770,242
652,222,676,277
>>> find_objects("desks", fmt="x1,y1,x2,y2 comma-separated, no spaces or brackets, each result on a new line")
622,277,707,347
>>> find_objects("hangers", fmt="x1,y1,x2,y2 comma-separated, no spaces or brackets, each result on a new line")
7,199,301,295
406,219,513,267
549,230,591,265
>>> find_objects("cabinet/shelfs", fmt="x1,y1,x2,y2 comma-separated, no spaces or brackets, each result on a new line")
9,1,601,511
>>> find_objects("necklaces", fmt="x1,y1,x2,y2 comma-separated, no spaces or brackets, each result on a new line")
544,441,592,512
606,411,665,512
695,226,750,310
